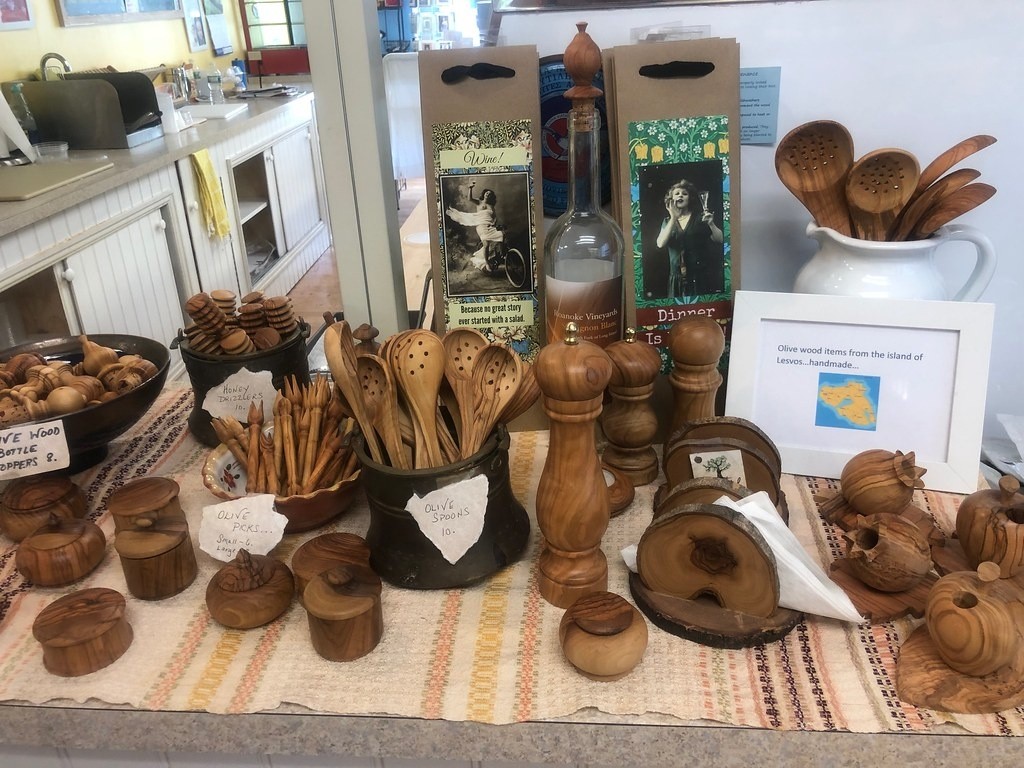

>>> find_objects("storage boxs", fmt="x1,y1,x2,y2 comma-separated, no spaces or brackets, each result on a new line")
247,44,310,76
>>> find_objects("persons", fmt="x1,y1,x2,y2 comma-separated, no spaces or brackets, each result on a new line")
440,17,448,31
657,178,723,298
447,181,504,272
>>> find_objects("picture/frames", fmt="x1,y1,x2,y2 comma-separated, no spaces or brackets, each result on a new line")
726,288,997,496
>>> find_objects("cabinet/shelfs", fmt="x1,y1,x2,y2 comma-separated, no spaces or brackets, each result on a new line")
0,82,334,387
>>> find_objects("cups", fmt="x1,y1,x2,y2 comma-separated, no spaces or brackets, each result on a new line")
113,514,199,601
303,565,384,663
173,68,188,99
107,477,187,537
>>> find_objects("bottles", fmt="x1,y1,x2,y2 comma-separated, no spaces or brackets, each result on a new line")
207,61,223,104
543,21,626,349
188,57,201,93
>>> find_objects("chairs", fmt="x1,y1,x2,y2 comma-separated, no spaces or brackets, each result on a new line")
381,50,430,211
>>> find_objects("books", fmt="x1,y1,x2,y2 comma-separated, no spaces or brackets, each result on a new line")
238,85,307,98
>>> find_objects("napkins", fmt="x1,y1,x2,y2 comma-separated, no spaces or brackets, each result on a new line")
619,489,868,625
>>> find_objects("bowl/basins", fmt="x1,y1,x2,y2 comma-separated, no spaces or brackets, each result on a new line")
200,421,361,533
0,335,172,477
30,140,69,164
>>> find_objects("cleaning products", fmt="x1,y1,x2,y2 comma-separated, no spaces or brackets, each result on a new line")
5,80,41,156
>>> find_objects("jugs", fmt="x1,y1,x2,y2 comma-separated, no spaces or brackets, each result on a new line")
793,218,998,302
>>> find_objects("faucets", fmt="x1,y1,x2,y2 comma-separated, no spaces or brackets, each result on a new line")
38,52,72,81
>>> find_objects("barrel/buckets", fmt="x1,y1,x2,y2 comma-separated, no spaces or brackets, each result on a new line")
350,412,531,590
168,322,311,448
